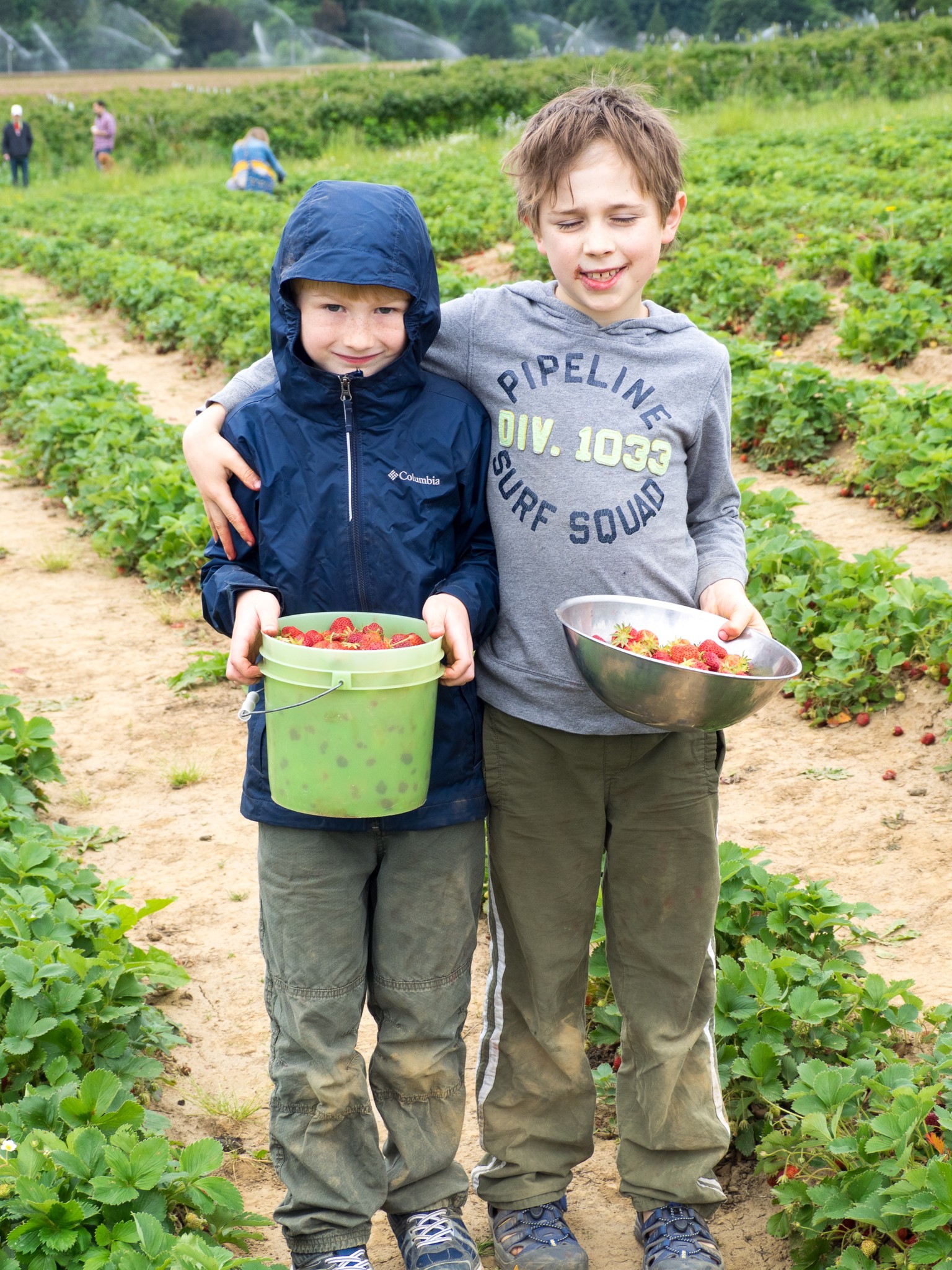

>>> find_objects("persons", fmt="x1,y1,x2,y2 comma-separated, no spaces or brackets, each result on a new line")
2,103,34,186
203,183,501,1270
87,101,114,167
179,86,774,1270
227,126,285,193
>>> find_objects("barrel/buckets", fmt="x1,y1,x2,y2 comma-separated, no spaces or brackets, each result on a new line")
238,609,446,819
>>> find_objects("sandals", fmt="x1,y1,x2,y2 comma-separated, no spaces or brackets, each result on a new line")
489,1192,591,1270
633,1206,725,1270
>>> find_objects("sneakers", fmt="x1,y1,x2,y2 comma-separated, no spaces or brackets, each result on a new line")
385,1206,485,1270
290,1244,373,1270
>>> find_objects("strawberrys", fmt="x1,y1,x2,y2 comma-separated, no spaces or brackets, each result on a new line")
613,1050,622,1070
587,994,592,1005
721,260,952,530
924,1112,941,1132
727,1103,782,1132
782,602,952,780
834,1218,922,1270
279,617,426,650
831,1157,843,1167
590,623,751,676
767,1164,798,1188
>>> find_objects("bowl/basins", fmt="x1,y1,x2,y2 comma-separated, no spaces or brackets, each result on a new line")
554,593,802,734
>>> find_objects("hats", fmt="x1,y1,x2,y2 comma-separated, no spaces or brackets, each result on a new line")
11,104,23,117
